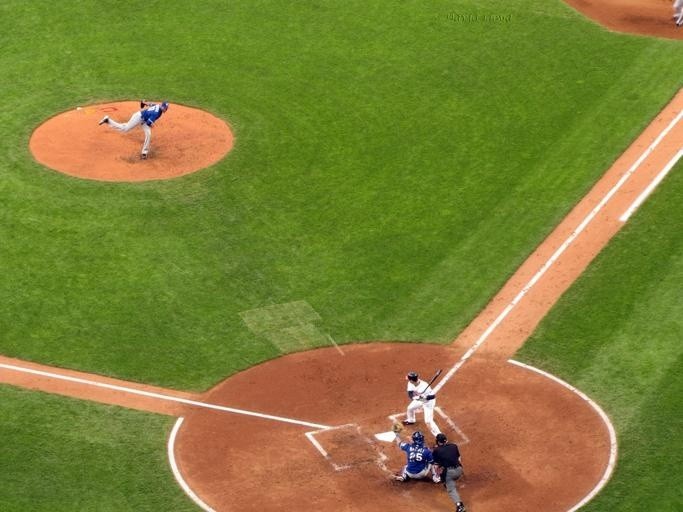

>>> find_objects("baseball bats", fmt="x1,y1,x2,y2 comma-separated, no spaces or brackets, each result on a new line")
421,369,443,394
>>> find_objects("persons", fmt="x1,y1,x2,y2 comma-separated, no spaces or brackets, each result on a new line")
392,423,441,483
432,434,465,511
403,372,441,437
674,0,683,26
99,102,169,158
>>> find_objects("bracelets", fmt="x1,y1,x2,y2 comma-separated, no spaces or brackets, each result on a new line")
396,434,400,438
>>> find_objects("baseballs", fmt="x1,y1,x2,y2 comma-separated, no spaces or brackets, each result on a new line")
77,107,83,111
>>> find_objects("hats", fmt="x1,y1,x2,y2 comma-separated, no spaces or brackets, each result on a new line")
436,434,447,445
404,371,418,383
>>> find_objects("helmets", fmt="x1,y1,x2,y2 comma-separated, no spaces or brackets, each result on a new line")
160,101,168,110
412,430,424,444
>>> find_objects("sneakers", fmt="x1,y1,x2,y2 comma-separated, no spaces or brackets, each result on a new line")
402,420,415,424
390,474,404,481
97,116,109,126
455,503,465,512
141,153,147,160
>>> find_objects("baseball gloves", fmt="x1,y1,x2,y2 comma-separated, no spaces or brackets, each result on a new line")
392,421,405,433
